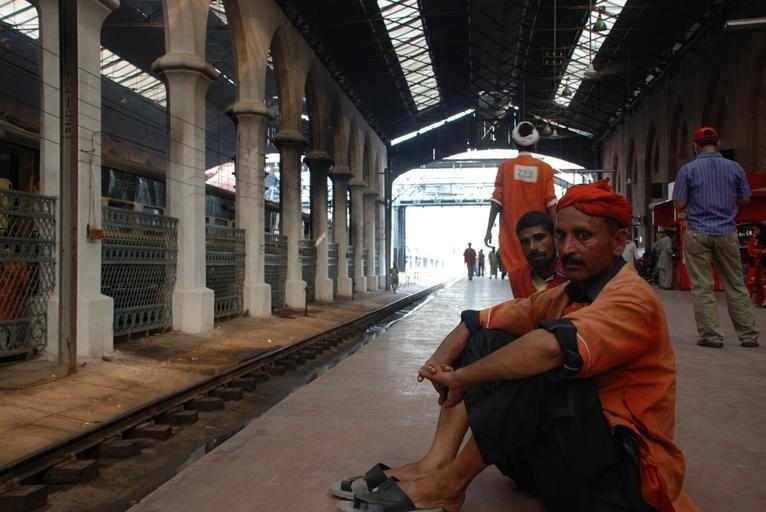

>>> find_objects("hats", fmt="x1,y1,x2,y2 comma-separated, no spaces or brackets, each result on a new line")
691,126,720,145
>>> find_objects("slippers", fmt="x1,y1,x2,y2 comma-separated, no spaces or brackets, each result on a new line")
335,476,445,511
330,463,393,501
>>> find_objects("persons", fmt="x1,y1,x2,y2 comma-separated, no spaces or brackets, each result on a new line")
463,242,507,280
388,261,399,294
650,229,677,289
0,243,30,347
622,234,641,267
484,119,559,274
508,209,571,300
744,225,766,299
672,127,761,347
330,174,700,511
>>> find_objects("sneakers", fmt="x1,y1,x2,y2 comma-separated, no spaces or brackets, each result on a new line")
741,340,760,347
697,338,724,348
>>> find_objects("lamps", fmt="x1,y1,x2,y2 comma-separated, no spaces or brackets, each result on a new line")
541,0,608,139
